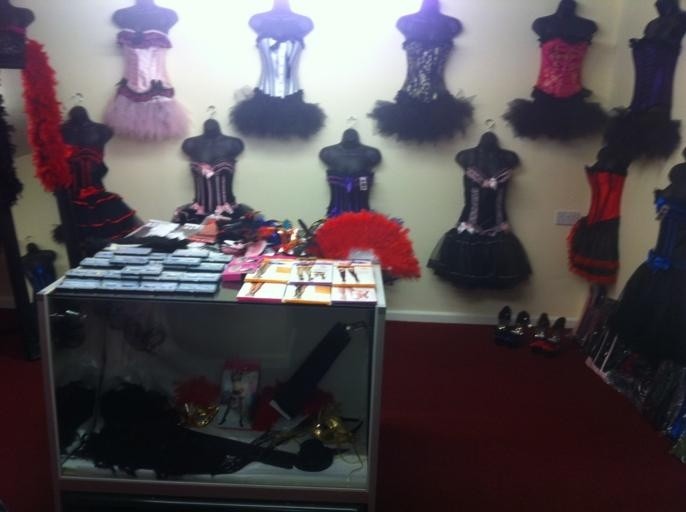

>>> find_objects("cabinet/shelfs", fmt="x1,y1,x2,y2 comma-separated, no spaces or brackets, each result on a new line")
36,245,387,512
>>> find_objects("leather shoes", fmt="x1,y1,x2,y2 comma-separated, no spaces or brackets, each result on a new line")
543,318,566,354
496,306,512,343
530,314,550,350
509,312,530,337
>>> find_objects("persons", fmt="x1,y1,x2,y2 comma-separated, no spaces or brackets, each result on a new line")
19,243,56,300
170,119,245,223
499,0,608,142
565,140,630,286
313,129,420,285
426,131,532,289
0,1,35,69
102,0,190,139
367,1,475,144
603,166,686,356
48,106,145,270
601,0,686,163
228,0,327,139
